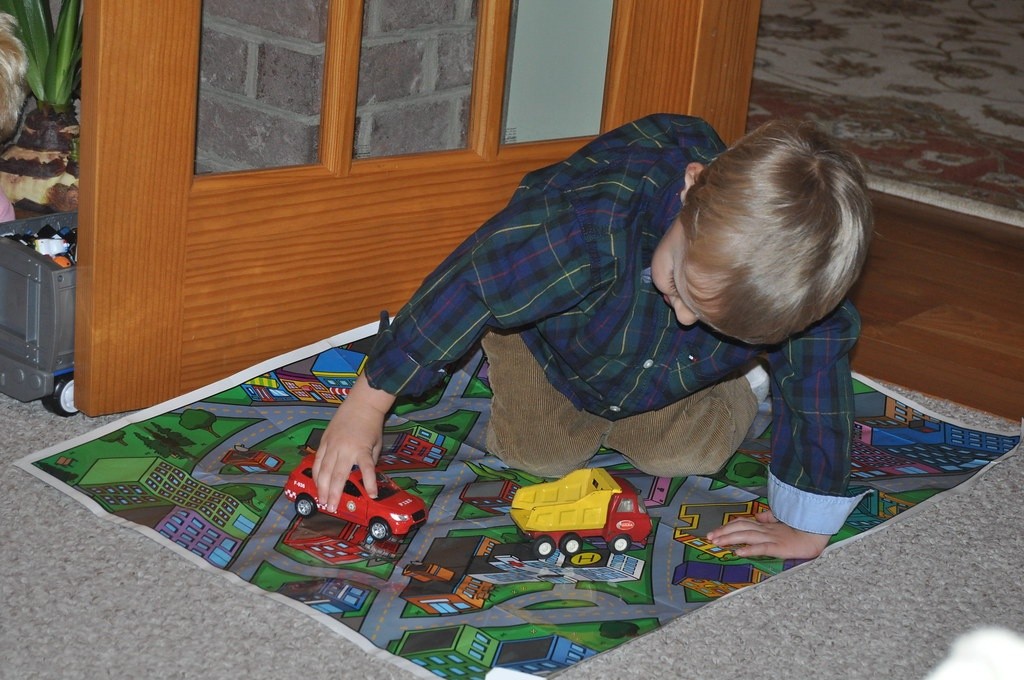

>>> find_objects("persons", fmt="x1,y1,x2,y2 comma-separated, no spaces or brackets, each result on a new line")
0,8,28,224
310,110,876,560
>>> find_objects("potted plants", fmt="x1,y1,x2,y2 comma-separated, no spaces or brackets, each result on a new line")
0,0,83,219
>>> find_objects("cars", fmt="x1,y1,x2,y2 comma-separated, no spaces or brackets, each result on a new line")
284,453,428,540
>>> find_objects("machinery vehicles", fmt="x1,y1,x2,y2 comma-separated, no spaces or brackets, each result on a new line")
510,466,652,557
